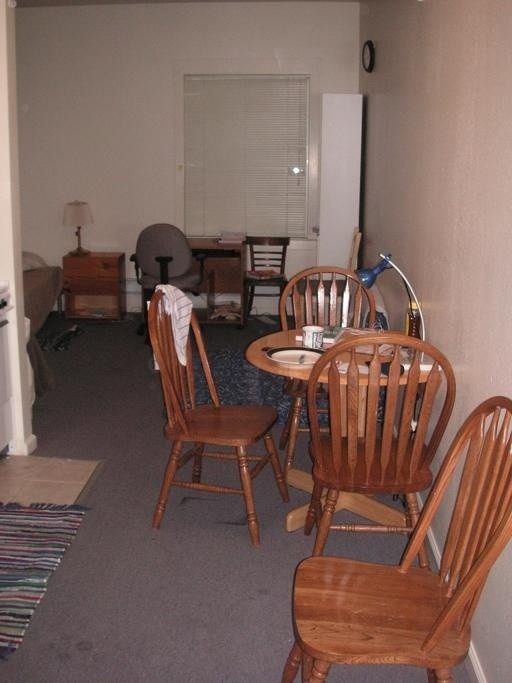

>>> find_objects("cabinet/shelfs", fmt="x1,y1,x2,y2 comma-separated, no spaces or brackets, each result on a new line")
61,247,128,322
311,88,369,281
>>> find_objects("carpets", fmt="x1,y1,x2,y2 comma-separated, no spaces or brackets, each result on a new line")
2,494,90,662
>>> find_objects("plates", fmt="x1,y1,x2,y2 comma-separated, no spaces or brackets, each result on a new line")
265,345,331,369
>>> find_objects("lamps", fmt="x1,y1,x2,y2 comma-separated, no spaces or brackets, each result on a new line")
61,197,97,257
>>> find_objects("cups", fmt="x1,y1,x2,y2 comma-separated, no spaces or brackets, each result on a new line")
302,326,324,351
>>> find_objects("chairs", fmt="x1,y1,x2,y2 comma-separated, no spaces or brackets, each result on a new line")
147,235,458,572
280,397,510,681
136,222,205,342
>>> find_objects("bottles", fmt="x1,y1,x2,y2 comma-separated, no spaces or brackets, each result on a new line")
405,300,422,341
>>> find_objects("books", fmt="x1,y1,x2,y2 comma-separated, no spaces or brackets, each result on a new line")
218,231,247,244
295,325,344,343
247,268,283,280
